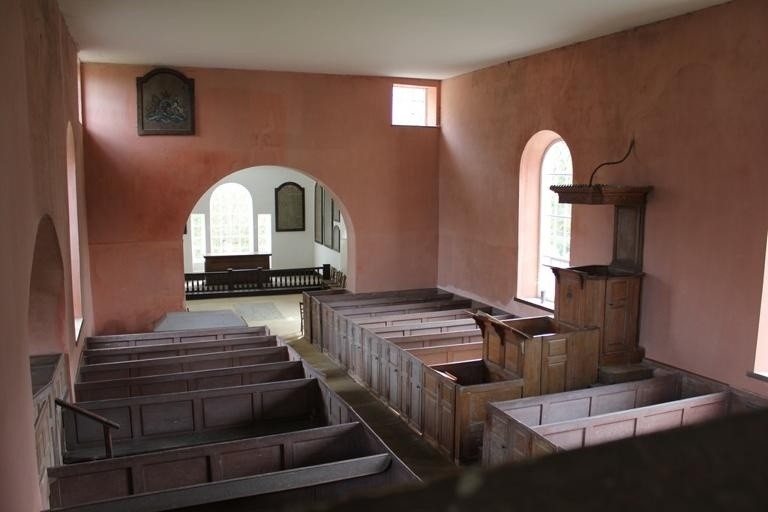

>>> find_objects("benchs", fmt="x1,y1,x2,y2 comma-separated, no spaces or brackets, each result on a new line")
44,325,424,511
302,287,730,469
